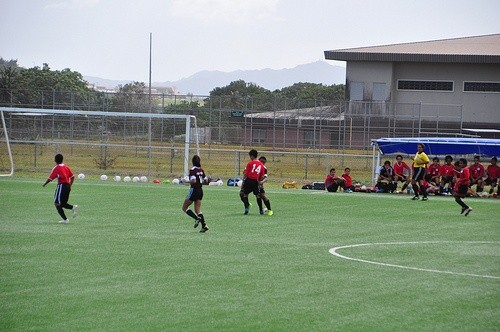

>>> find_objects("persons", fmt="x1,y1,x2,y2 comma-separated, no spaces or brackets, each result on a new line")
411,143,431,201
181,155,208,233
375,154,500,198
43,153,77,224
325,168,371,193
240,150,272,215
453,158,472,216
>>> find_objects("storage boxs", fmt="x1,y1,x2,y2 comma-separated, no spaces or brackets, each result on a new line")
314,183,325,190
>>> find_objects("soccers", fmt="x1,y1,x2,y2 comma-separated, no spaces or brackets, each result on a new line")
237,181,242,187
173,179,179,184
113,176,121,182
133,176,139,182
184,176,189,182
141,176,148,182
101,175,107,180
78,173,85,180
124,176,131,182
217,180,223,186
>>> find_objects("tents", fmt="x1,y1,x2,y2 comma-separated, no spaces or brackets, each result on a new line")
371,136,500,186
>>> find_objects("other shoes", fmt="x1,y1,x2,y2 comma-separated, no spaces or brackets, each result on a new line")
447,192,452,196
260,211,263,215
393,190,396,194
244,209,248,215
489,189,493,194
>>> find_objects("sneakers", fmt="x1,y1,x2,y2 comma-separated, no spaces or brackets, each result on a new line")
59,219,69,224
194,217,202,228
411,196,420,200
267,210,273,216
421,197,428,201
345,189,352,193
200,226,208,233
465,207,472,216
460,206,466,214
397,190,403,194
71,205,78,218
